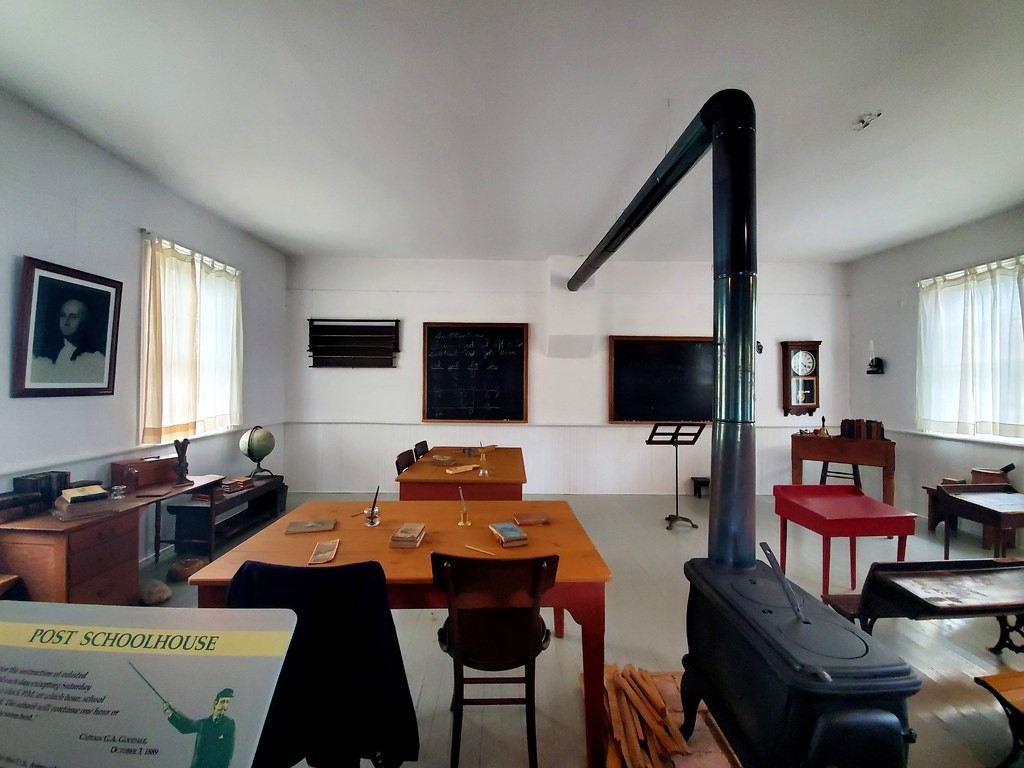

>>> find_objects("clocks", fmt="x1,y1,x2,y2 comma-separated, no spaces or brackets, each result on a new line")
781,340,822,416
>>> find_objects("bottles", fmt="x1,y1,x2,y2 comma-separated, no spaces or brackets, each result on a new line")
125,464,139,497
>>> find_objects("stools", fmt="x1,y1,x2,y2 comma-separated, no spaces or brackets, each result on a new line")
820,461,862,488
692,476,709,499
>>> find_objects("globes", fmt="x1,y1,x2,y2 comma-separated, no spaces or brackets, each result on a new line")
239,426,276,479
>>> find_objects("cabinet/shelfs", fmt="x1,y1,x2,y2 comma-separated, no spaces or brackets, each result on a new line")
167,473,289,551
307,318,400,369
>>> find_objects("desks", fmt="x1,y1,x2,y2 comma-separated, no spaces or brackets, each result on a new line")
773,484,917,605
790,429,895,539
396,448,527,502
935,481,1024,560
188,502,612,768
0,473,225,603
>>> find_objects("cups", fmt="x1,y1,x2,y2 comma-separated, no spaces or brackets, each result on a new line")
111,485,126,500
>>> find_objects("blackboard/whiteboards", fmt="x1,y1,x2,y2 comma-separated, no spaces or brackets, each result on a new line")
422,323,529,423
609,335,715,425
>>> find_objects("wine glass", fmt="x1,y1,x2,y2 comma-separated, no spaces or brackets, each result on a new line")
363,506,379,526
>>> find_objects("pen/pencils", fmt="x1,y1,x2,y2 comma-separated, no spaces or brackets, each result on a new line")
351,511,364,517
370,485,380,516
465,545,495,556
459,486,466,511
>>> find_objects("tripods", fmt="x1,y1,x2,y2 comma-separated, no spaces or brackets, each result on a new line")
645,423,708,530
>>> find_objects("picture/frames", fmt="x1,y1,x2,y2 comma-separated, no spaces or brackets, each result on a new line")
11,255,124,393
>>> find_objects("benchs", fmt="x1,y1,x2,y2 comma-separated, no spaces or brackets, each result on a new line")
822,557,1024,655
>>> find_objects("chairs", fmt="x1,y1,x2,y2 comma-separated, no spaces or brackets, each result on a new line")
414,439,429,461
427,550,560,768
395,449,416,475
